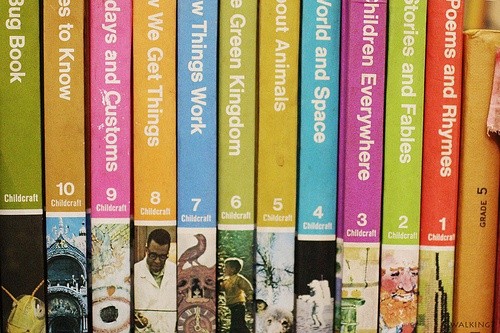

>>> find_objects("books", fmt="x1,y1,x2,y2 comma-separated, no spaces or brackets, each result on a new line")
0,0,500,333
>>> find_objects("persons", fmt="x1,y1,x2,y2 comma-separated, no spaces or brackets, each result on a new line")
219,258,254,333
297,280,327,330
134,229,176,333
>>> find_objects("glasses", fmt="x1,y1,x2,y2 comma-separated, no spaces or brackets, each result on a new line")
147,253,168,262
